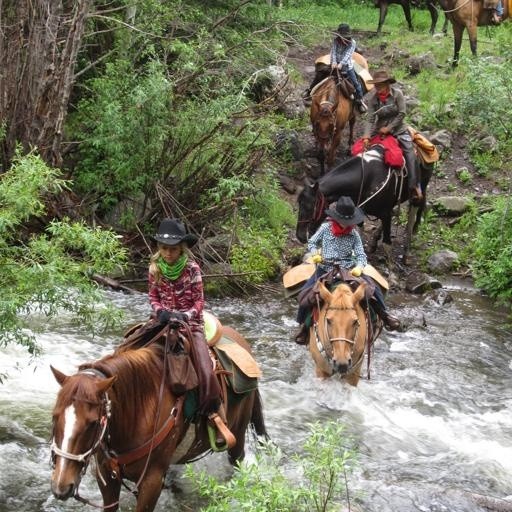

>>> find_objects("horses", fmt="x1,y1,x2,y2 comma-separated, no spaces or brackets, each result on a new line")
375,0,451,37
309,76,359,178
50,310,269,511
307,281,371,386
294,134,435,278
431,0,512,69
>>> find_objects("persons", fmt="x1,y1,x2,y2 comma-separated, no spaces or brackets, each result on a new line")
292,195,401,343
362,68,425,203
488,0,506,21
303,22,369,114
115,214,232,449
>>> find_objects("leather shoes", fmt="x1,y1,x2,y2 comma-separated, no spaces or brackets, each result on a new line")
410,190,420,204
214,427,228,448
384,317,407,332
295,325,309,345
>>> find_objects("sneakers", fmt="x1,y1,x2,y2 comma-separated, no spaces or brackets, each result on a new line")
357,99,368,113
301,89,312,99
491,14,502,26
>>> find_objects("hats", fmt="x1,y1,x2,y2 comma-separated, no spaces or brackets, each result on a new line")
147,217,199,247
331,23,354,38
365,70,397,85
324,196,364,226
